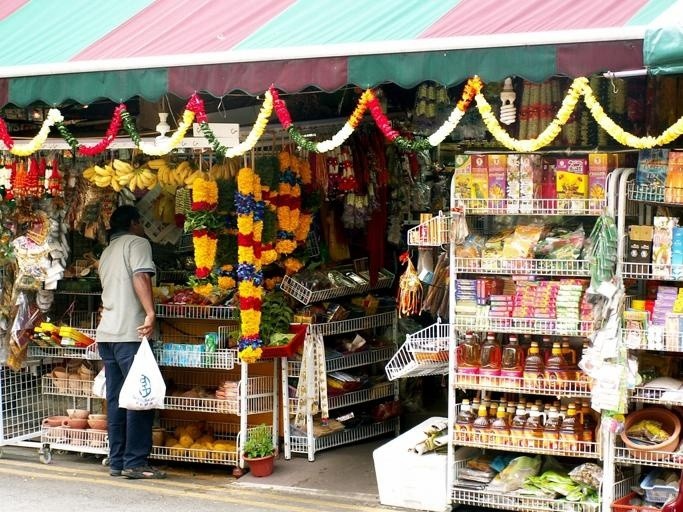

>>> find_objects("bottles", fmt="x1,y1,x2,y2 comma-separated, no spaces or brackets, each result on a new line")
456,331,593,390
454,395,595,451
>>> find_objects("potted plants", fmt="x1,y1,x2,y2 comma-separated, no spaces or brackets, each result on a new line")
240,421,276,477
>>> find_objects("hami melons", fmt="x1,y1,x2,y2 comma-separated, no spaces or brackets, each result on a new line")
165,422,237,460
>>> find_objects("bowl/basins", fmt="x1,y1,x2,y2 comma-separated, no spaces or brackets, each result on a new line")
47,359,96,396
619,407,682,462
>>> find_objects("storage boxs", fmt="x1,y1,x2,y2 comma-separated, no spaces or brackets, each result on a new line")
372,413,447,510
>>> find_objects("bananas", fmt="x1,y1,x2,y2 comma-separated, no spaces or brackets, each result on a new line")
83,159,240,193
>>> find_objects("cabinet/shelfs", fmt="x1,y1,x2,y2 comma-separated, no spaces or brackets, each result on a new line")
383,203,448,387
28,302,279,476
440,147,626,511
605,165,682,512
279,256,400,460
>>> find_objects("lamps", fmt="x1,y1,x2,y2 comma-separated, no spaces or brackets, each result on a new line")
153,96,171,156
497,75,517,126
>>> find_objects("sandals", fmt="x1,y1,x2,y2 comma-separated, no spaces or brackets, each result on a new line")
109,467,123,477
120,462,169,480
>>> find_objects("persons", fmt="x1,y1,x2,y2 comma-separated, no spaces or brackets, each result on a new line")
96,204,167,480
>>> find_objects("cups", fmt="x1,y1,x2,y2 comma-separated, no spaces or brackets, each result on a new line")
39,408,108,446
